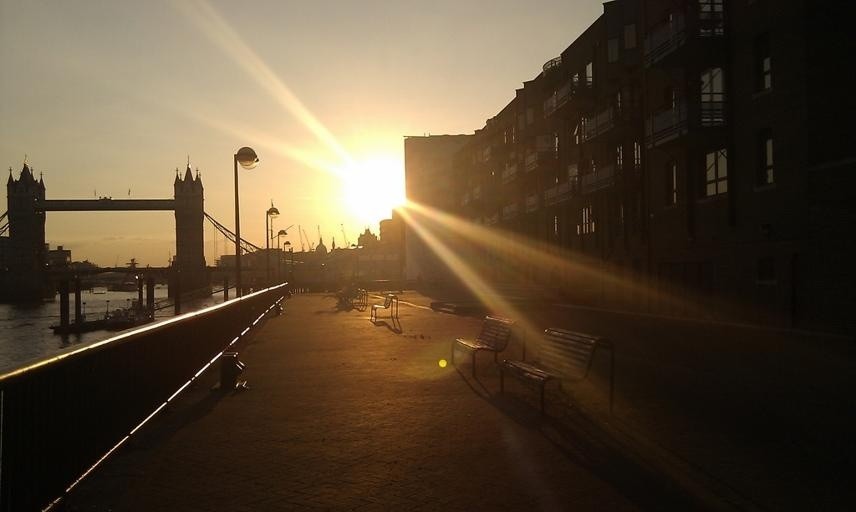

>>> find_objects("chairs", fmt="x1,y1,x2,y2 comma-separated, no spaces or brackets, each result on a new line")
451,313,616,418
371,294,398,322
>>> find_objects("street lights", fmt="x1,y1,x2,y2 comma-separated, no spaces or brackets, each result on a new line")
105,299,110,316
82,301,87,319
266,203,280,288
233,146,258,296
284,241,290,283
278,229,287,284
